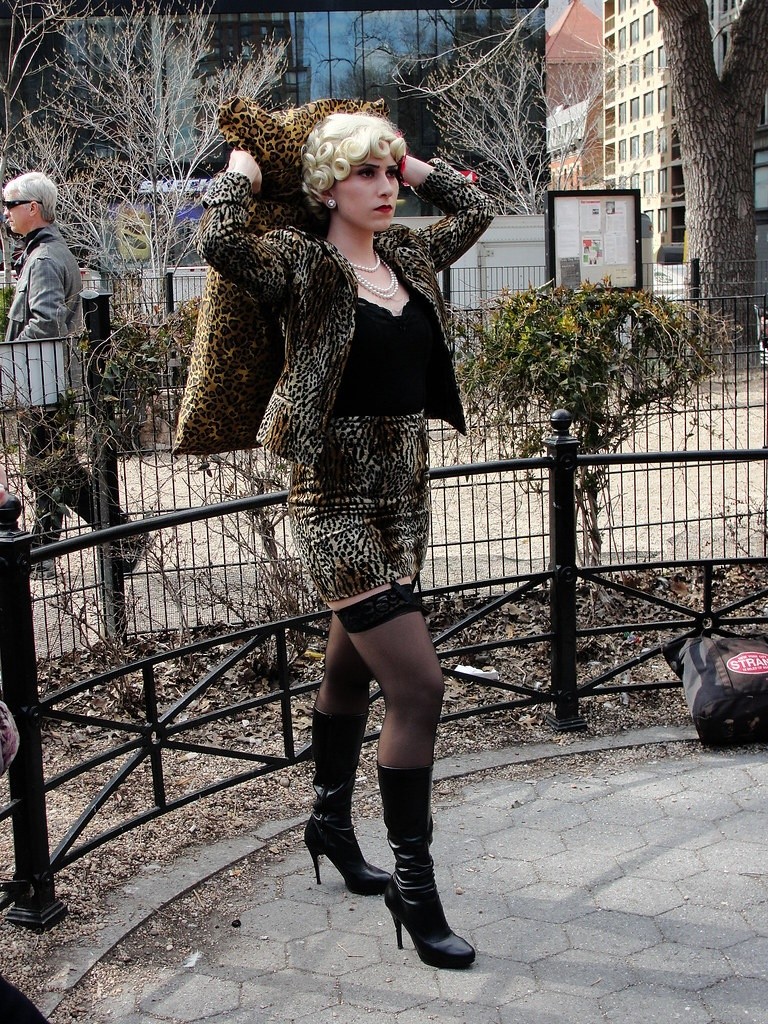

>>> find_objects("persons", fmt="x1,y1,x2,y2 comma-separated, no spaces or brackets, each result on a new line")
2,170,156,583
196,109,495,970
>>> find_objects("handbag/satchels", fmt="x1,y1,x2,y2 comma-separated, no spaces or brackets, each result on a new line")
661,634,768,745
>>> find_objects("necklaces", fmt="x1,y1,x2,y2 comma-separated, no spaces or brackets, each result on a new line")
345,247,399,300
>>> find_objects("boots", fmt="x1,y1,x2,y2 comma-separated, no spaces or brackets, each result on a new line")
375,759,476,970
304,708,392,896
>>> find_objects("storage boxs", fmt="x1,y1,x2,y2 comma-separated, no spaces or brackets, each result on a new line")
662,637,768,747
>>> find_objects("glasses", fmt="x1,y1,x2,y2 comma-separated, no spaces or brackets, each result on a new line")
2,200,42,211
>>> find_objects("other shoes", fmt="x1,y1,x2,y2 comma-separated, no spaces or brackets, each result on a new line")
30,560,61,580
121,531,155,578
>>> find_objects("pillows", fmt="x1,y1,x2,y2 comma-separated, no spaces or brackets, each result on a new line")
172,95,390,455
